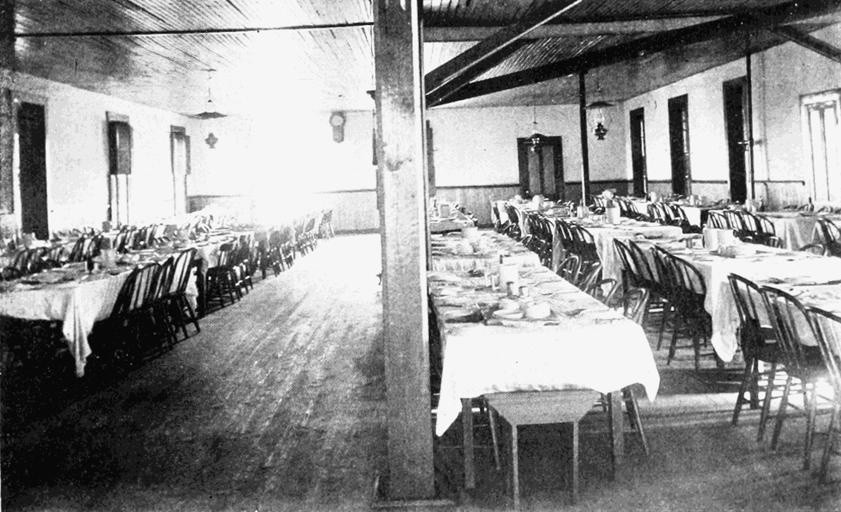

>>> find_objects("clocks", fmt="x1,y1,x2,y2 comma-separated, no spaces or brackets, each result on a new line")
329,111,346,143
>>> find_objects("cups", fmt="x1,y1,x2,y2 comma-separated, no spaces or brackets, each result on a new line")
605,207,621,225
482,263,519,291
702,228,734,251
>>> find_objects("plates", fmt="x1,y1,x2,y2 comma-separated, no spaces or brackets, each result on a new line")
491,301,552,321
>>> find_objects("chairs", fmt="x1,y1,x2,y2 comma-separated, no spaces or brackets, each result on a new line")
1,206,335,404
428,196,660,493
489,187,841,512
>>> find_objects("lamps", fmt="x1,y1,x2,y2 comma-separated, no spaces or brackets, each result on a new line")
527,95,545,155
188,69,229,149
580,69,616,141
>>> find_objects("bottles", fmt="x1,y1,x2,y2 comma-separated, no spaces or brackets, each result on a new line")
507,282,529,298
